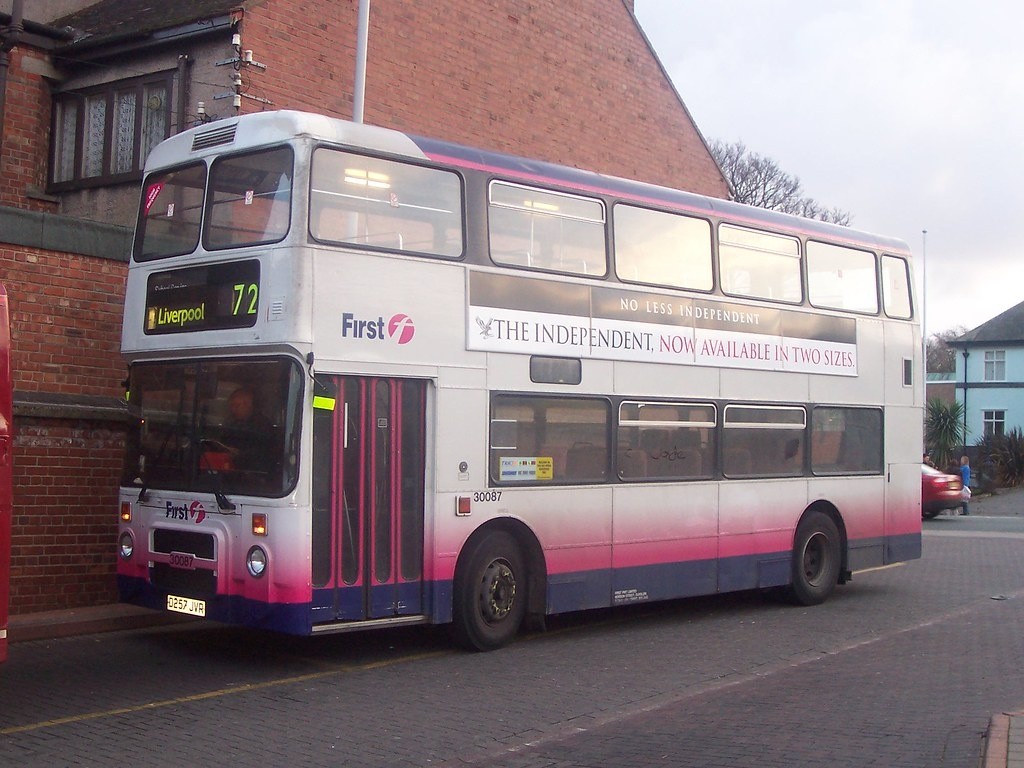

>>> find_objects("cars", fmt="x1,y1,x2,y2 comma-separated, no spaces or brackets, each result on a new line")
919,462,969,522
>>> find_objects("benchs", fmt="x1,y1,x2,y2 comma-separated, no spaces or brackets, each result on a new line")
567,426,847,479
339,232,462,256
492,250,588,274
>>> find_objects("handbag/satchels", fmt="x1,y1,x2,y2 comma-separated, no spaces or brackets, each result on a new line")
960,485,972,503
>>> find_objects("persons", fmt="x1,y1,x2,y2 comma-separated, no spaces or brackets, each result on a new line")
944,459,963,516
224,388,282,495
923,454,930,465
960,456,971,515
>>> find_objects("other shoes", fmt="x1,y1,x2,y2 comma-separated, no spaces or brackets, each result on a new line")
960,512,969,516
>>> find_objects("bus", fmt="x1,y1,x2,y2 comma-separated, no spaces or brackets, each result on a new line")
113,108,926,659
